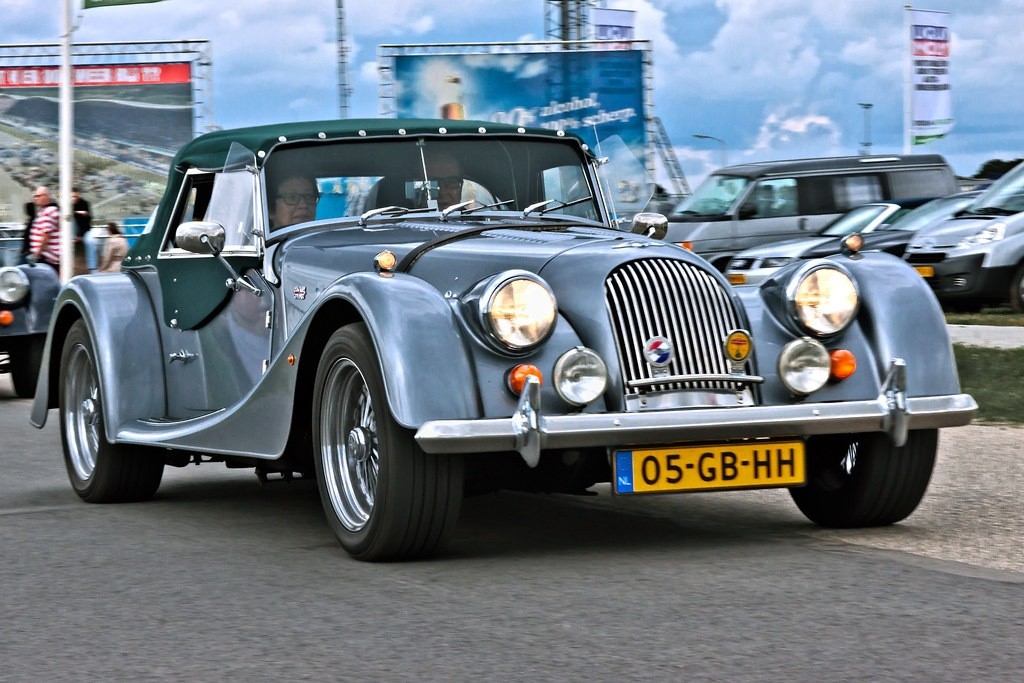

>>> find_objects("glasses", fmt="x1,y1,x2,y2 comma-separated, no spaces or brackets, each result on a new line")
273,193,321,206
425,177,464,189
33,194,48,198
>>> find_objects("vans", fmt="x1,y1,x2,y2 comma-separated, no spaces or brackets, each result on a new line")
658,152,958,276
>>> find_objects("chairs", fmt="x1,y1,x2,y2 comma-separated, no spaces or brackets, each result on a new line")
363,176,420,216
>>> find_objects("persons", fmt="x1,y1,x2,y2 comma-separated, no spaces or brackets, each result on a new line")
18,187,91,275
412,146,464,210
752,186,777,217
268,172,321,233
98,223,128,273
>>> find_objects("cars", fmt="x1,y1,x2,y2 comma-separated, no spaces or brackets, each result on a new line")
723,196,941,287
903,160,1024,316
31,119,979,564
0,247,64,401
795,188,988,259
